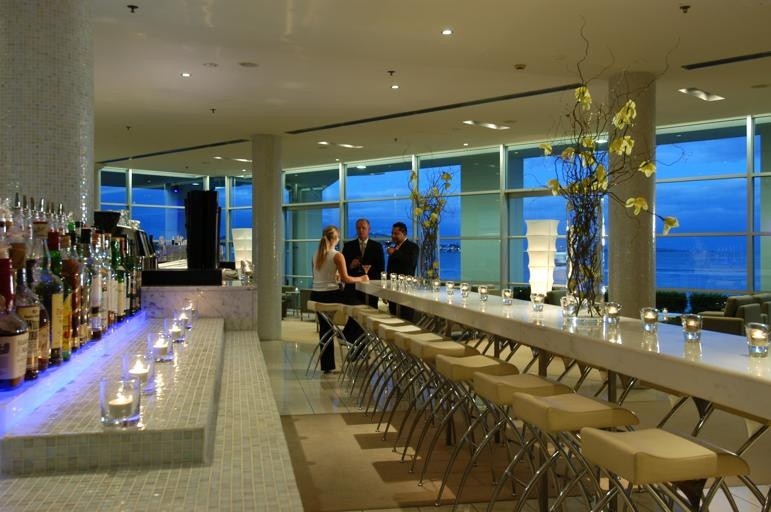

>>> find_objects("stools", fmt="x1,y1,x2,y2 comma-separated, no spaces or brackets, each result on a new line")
549,426,751,512
486,391,639,512
433,371,592,512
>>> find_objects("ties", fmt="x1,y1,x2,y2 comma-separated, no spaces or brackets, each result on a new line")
360,240,365,258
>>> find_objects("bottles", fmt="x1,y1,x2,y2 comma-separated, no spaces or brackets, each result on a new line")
0,192,142,386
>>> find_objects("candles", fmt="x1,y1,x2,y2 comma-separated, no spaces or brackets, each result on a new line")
108,391,133,421
153,302,199,362
128,360,149,387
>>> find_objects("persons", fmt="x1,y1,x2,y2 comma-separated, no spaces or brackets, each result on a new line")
310,225,370,374
382,222,419,322
342,219,385,309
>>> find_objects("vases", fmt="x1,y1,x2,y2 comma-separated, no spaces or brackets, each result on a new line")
565,199,604,318
420,228,439,289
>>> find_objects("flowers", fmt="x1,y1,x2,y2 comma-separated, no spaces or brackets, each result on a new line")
403,168,456,289
522,14,684,317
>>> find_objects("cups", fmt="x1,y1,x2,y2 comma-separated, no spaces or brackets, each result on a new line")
559,296,578,318
380,272,419,288
641,307,658,331
680,314,702,342
432,278,513,305
745,323,770,358
530,292,543,312
99,297,200,432
605,303,622,324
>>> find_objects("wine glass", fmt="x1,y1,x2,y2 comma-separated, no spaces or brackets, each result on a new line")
241,261,255,287
362,265,372,275
384,241,392,257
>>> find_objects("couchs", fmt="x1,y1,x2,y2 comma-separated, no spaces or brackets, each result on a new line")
698,295,761,337
753,293,771,324
